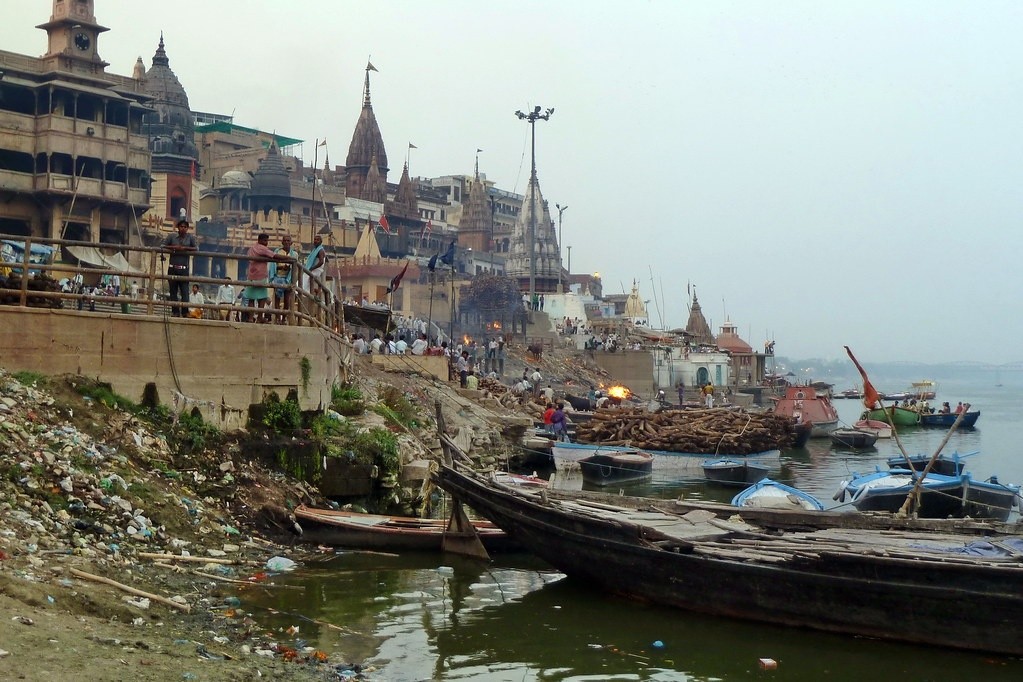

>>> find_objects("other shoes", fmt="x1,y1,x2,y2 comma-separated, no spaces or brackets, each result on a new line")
171,313,180,317
246,319,254,322
256,317,267,323
282,320,286,325
275,319,280,325
183,313,195,318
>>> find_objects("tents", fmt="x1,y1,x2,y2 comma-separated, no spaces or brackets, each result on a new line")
66,245,143,294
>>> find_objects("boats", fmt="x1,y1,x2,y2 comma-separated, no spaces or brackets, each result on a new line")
950,475,1020,522
864,405,921,428
427,458,1023,657
855,419,893,439
833,388,936,401
920,410,981,429
577,448,654,487
772,377,839,439
700,457,771,484
828,426,879,449
886,452,967,477
844,463,968,518
293,505,530,554
730,477,824,512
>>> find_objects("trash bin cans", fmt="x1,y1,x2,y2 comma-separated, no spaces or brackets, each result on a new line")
121,303,131,314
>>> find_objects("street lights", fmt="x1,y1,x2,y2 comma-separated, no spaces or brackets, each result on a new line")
556,203,568,284
514,105,555,308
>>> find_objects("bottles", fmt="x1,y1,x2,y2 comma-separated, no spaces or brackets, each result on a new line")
438,566,454,574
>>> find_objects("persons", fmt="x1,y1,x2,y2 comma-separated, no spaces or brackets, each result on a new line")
410,333,428,356
550,402,568,442
163,218,198,317
216,276,235,320
540,294,544,311
703,382,714,409
38,267,139,311
457,350,470,388
655,388,666,405
530,367,543,396
188,233,969,444
489,337,498,358
296,235,326,296
679,382,684,405
179,206,187,221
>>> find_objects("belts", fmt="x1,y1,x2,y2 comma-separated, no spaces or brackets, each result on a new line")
169,264,189,269
220,302,232,304
